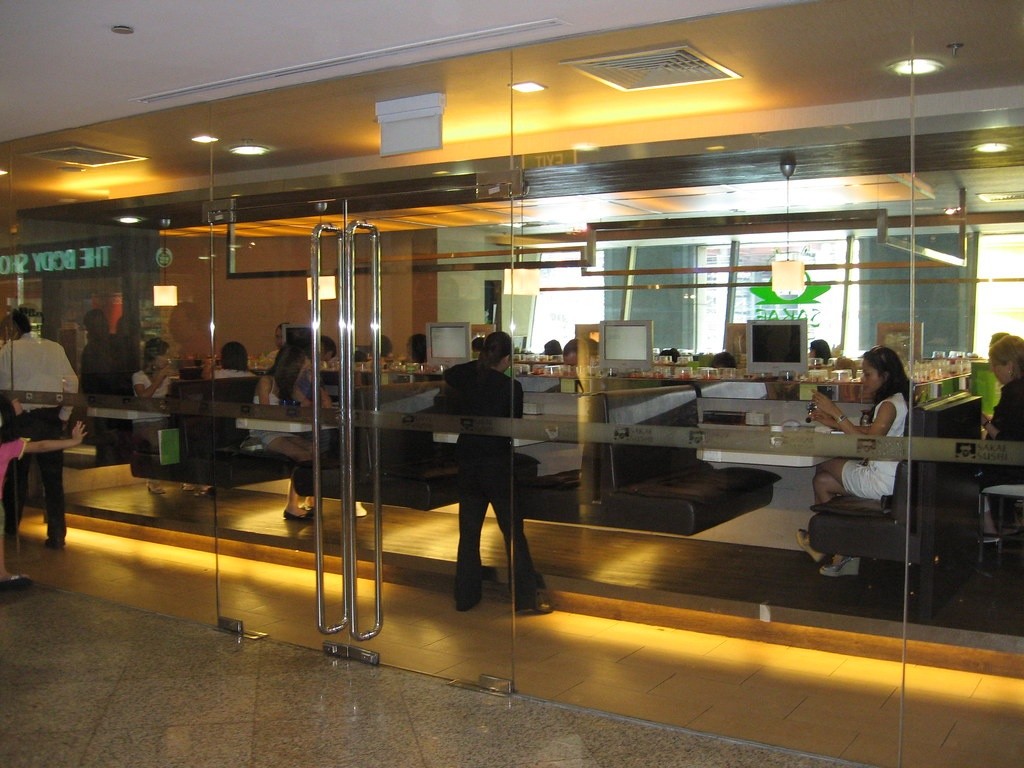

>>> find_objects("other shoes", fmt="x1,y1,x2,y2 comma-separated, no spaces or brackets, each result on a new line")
456,594,482,611
284,504,315,519
515,600,554,614
0,575,31,590
45,537,66,547
355,501,368,517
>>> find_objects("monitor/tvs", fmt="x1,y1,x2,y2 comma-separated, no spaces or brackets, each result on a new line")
600,321,653,371
426,322,472,367
746,319,808,377
282,324,312,360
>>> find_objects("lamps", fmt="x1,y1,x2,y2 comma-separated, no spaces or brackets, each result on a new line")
771,164,806,300
307,203,336,300
153,218,177,306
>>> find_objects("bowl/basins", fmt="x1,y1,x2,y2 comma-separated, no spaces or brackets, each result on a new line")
653,347,736,379
808,358,862,382
179,367,202,380
514,353,618,377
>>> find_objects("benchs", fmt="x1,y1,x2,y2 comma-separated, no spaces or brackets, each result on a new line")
81,375,1024,620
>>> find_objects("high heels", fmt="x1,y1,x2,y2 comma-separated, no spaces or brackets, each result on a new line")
194,486,217,497
147,480,167,494
795,529,827,562
181,483,194,491
820,555,859,575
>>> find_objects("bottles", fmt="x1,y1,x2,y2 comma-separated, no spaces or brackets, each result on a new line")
359,364,369,385
380,364,388,385
195,353,212,380
902,351,979,403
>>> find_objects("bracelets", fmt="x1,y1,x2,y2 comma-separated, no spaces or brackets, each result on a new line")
835,415,847,424
983,420,992,427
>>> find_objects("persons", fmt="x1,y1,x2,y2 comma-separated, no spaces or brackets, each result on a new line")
471,337,484,352
167,304,210,384
711,352,737,369
193,341,256,497
547,337,599,393
248,334,367,520
132,338,194,494
809,340,831,365
0,310,79,549
796,345,916,577
355,335,393,387
79,309,138,464
442,331,556,614
659,349,701,398
407,334,443,383
0,414,87,582
979,334,1024,543
267,322,290,359
544,340,562,355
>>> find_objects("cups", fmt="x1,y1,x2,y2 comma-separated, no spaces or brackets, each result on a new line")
280,399,300,407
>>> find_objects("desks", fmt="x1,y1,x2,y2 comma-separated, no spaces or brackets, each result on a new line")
87,408,172,421
235,417,336,433
695,428,831,466
432,431,543,447
